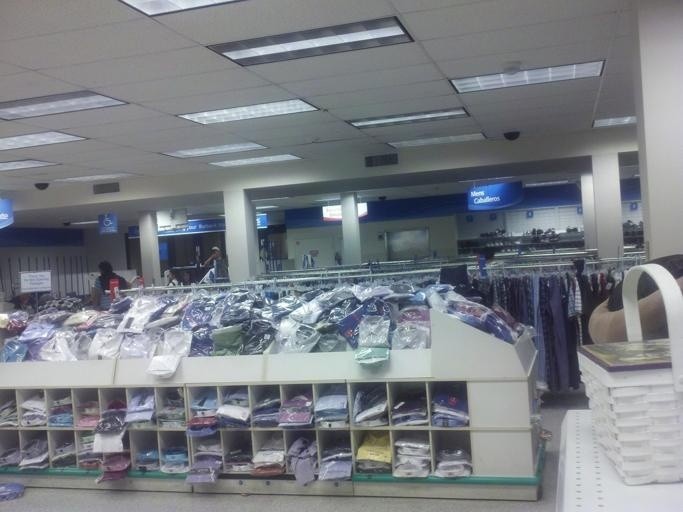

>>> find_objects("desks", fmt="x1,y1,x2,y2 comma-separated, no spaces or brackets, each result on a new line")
557,406,682,509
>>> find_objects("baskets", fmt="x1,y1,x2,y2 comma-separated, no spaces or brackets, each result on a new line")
577,264,683,486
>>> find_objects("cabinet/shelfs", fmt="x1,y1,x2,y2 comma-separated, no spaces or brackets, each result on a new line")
0,298,547,499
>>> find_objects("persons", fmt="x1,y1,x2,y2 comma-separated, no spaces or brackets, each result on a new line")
93,261,140,309
260,236,272,271
588,254,682,344
334,252,341,265
10,295,35,306
204,247,222,270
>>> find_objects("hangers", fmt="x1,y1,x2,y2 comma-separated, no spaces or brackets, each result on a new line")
458,253,644,284
